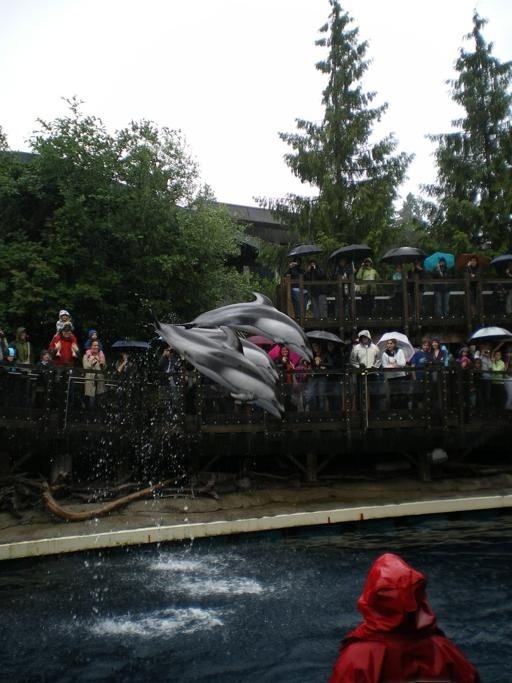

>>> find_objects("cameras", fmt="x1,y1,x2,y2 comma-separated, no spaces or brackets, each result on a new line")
439,264,443,267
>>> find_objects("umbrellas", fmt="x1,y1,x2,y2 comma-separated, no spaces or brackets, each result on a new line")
381,246,427,275
245,336,276,348
466,325,512,349
456,252,491,277
423,250,456,275
267,343,302,369
285,244,324,279
108,339,152,367
375,331,416,363
303,330,345,356
486,254,511,275
328,242,373,275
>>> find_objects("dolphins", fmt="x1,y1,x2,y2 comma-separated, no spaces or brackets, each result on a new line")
141,291,315,418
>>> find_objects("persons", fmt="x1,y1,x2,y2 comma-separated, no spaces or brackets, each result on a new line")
113,350,135,378
0,324,35,395
333,252,360,321
153,344,199,391
349,328,383,412
429,256,453,319
46,321,80,398
330,550,482,682
280,257,308,321
271,346,297,403
305,259,331,319
35,349,52,366
54,309,80,359
83,329,105,368
355,256,380,320
503,262,512,317
461,255,483,317
386,261,410,320
408,336,511,416
297,341,351,419
82,339,107,413
381,339,407,414
404,259,426,322
